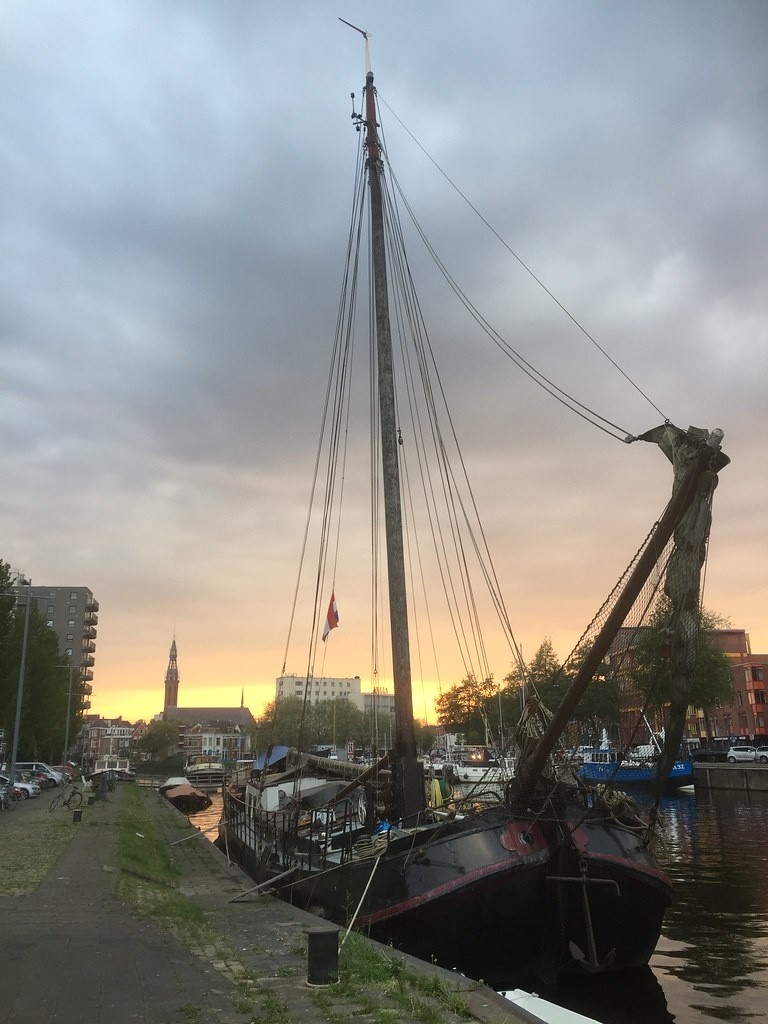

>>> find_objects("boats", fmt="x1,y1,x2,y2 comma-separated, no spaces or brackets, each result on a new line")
183,754,229,795
415,712,518,785
158,775,213,808
574,708,698,794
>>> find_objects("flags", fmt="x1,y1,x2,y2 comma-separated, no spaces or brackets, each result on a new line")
322,591,339,642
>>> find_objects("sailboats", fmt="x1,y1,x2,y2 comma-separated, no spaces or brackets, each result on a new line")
209,16,732,979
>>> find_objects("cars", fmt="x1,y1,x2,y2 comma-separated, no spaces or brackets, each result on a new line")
555,745,768,766
0,762,74,799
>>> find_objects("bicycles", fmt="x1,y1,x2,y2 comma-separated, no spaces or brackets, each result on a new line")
0,782,18,812
49,778,83,813
